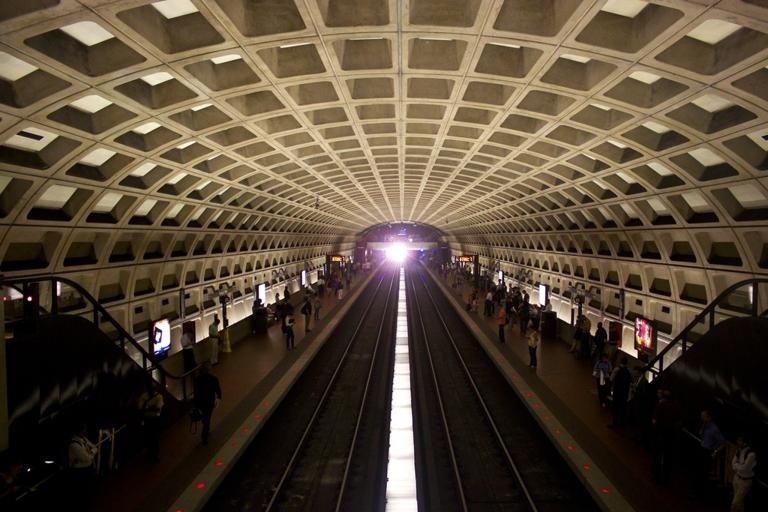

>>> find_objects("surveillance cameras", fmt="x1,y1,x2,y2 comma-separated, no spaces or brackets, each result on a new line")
314,202,319,208
445,219,449,224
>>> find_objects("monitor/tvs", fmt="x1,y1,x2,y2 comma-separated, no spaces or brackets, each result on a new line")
300,270,306,286
498,270,504,285
538,285,548,307
148,316,172,355
633,316,657,356
256,283,266,305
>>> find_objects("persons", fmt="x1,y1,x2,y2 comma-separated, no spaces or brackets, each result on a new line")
695,408,724,496
728,435,757,512
594,353,682,430
181,331,196,373
209,318,220,366
591,322,608,359
67,423,100,485
568,314,590,353
523,325,538,368
252,259,372,351
137,381,164,469
0,448,23,512
438,260,551,343
191,358,223,444
